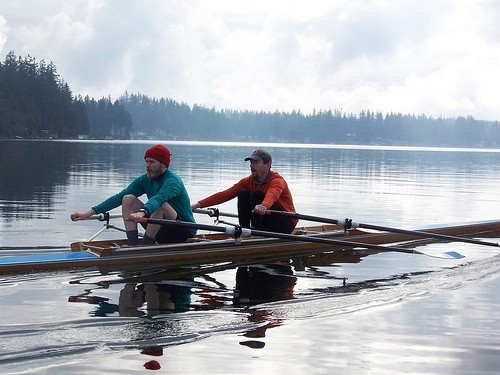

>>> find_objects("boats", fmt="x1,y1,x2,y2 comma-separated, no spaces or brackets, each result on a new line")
0,210,500,276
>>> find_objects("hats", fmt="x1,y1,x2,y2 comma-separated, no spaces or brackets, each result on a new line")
245,149,271,162
144,144,171,167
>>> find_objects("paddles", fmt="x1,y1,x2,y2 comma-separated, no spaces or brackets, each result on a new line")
73,213,123,220
255,209,500,248
193,207,238,218
130,216,465,259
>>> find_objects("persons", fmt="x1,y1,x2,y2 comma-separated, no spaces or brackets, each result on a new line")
69,264,192,370
70,143,198,245
191,149,300,234
231,259,298,349
